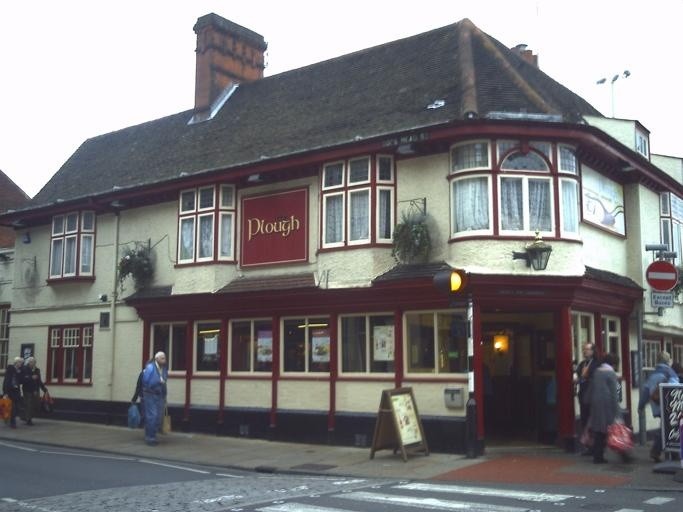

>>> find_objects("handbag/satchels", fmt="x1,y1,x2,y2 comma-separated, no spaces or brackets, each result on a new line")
579,431,595,450
0,398,12,420
162,415,171,436
42,391,54,414
607,424,632,450
652,378,667,402
128,405,141,428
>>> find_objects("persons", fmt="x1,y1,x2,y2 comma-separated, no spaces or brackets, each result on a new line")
582,352,638,463
131,359,153,415
141,351,168,444
637,351,681,463
575,342,600,455
3,356,26,429
19,356,49,425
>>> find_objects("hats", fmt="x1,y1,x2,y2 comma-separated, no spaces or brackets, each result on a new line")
155,351,167,365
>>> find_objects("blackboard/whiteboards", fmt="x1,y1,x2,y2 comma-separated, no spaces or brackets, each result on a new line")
658,382,683,452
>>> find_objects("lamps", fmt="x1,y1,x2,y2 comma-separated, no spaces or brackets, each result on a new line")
512,229,552,269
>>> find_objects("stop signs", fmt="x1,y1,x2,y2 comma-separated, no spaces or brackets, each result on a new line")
646,261,677,292
